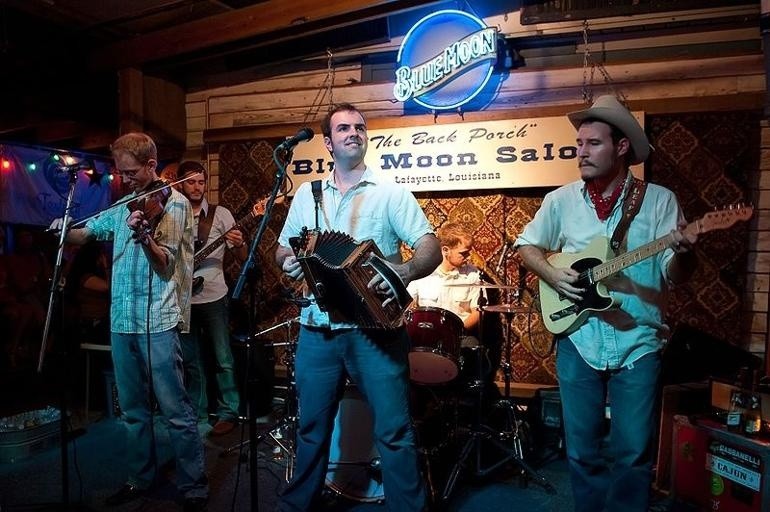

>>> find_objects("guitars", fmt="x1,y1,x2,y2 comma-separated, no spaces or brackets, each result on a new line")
192,189,284,292
538,200,754,337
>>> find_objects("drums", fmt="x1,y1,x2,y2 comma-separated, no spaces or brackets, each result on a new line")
405,306,463,386
325,379,443,502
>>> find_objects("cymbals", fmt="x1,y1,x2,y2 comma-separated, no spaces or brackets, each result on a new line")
482,303,539,313
443,281,521,289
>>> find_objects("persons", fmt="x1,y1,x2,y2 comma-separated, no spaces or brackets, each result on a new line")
512,93,700,511
47,132,212,511
400,220,491,342
175,161,251,437
274,103,443,512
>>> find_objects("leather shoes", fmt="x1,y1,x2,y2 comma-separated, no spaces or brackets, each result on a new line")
104,484,138,502
184,497,208,512
211,421,234,434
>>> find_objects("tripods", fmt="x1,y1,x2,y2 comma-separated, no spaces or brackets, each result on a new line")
218,326,298,484
493,315,533,489
441,297,558,502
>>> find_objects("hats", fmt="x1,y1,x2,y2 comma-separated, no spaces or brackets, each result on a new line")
568,95,649,165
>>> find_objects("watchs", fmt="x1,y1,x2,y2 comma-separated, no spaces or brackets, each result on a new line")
237,239,247,250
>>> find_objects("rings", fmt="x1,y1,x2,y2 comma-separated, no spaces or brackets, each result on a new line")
674,241,679,248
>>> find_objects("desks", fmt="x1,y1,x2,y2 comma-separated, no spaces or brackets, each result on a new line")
79,341,113,432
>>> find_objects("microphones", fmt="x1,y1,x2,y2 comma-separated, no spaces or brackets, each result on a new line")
496,239,512,273
58,159,94,172
278,128,315,152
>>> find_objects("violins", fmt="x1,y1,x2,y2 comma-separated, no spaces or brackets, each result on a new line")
127,180,171,246
